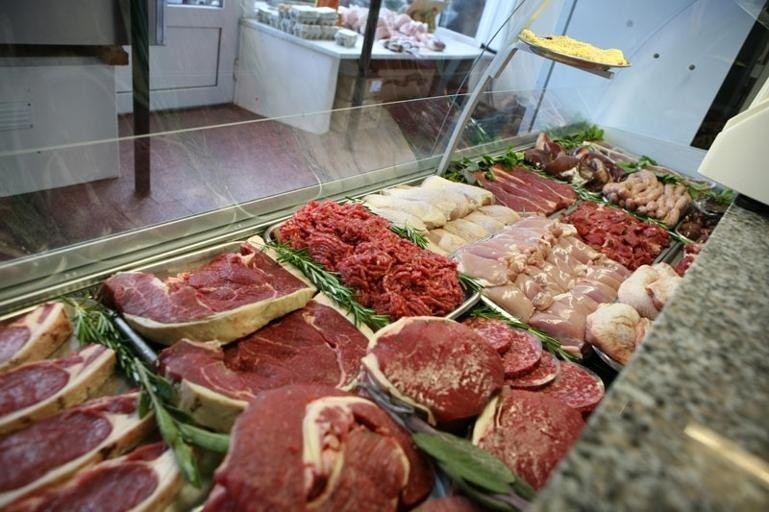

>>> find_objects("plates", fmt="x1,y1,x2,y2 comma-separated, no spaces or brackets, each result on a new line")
517,34,632,71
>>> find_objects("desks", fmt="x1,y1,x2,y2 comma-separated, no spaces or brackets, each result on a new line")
234,18,496,135
